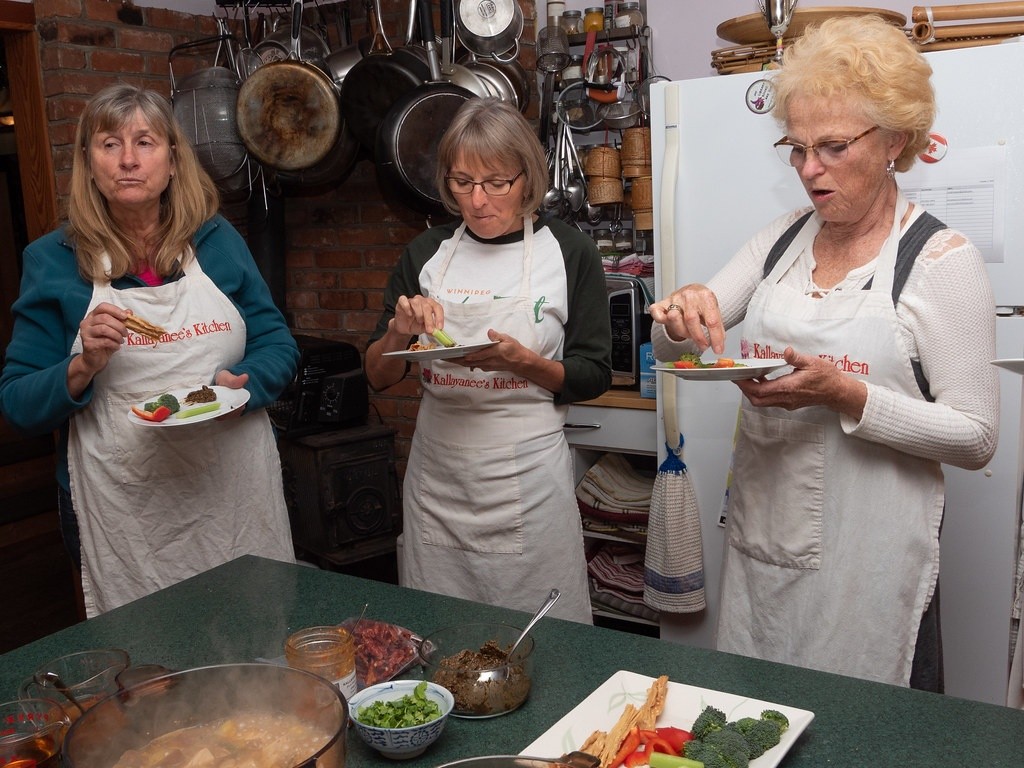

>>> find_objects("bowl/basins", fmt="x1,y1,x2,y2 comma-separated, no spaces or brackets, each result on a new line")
421,621,535,720
59,662,349,768
348,680,454,758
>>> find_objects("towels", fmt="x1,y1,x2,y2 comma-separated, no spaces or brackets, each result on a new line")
585,546,660,624
573,452,656,545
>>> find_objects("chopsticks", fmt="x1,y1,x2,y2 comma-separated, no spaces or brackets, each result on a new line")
515,670,814,768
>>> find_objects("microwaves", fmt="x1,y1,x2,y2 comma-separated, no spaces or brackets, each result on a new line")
606,273,653,387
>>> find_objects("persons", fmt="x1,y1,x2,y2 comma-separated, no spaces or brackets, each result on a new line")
0,84,302,619
648,10,1001,698
362,95,613,627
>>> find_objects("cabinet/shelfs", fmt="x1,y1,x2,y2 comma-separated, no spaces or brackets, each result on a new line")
537,25,651,135
567,404,656,629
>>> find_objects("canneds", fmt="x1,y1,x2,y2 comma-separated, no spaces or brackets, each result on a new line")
283,626,357,712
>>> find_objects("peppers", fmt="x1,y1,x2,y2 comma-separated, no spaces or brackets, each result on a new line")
674,357,734,369
606,724,695,768
131,406,171,422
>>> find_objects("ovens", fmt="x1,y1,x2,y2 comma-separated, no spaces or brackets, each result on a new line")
260,334,369,437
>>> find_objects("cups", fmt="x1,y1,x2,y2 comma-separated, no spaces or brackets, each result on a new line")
562,7,604,35
547,2,566,37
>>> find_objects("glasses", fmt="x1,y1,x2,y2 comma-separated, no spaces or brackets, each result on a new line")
773,124,881,167
443,169,523,196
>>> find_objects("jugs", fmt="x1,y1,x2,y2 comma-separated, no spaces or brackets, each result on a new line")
0,700,72,768
19,648,188,749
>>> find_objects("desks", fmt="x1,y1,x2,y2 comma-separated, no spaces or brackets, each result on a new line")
278,422,403,573
0,554,1023,768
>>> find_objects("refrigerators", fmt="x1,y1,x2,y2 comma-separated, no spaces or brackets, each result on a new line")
650,43,1024,706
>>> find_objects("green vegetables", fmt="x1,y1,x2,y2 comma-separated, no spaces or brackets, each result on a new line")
354,680,442,729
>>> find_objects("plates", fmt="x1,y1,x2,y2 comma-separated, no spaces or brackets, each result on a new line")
648,360,787,380
128,385,251,426
649,358,788,381
381,340,501,363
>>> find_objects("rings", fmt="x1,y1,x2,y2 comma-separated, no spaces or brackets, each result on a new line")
667,304,683,314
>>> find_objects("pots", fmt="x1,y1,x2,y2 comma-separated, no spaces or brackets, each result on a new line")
434,752,600,768
236,0,345,178
340,1,529,202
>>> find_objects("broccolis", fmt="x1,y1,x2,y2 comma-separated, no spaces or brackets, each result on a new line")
683,705,789,768
679,351,702,369
144,393,180,414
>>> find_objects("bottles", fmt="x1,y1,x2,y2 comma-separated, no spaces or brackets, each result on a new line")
286,626,358,727
614,2,644,29
562,55,584,87
582,229,645,258
